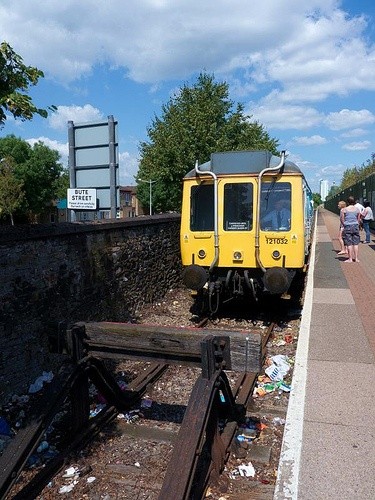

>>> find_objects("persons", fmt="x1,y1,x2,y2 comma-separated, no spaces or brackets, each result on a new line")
337,200,350,255
359,199,375,244
339,195,361,263
262,197,291,231
354,198,366,229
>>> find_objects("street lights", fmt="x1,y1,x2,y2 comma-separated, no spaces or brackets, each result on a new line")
138,178,156,215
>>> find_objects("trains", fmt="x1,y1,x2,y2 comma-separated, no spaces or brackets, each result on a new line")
179,150,314,325
